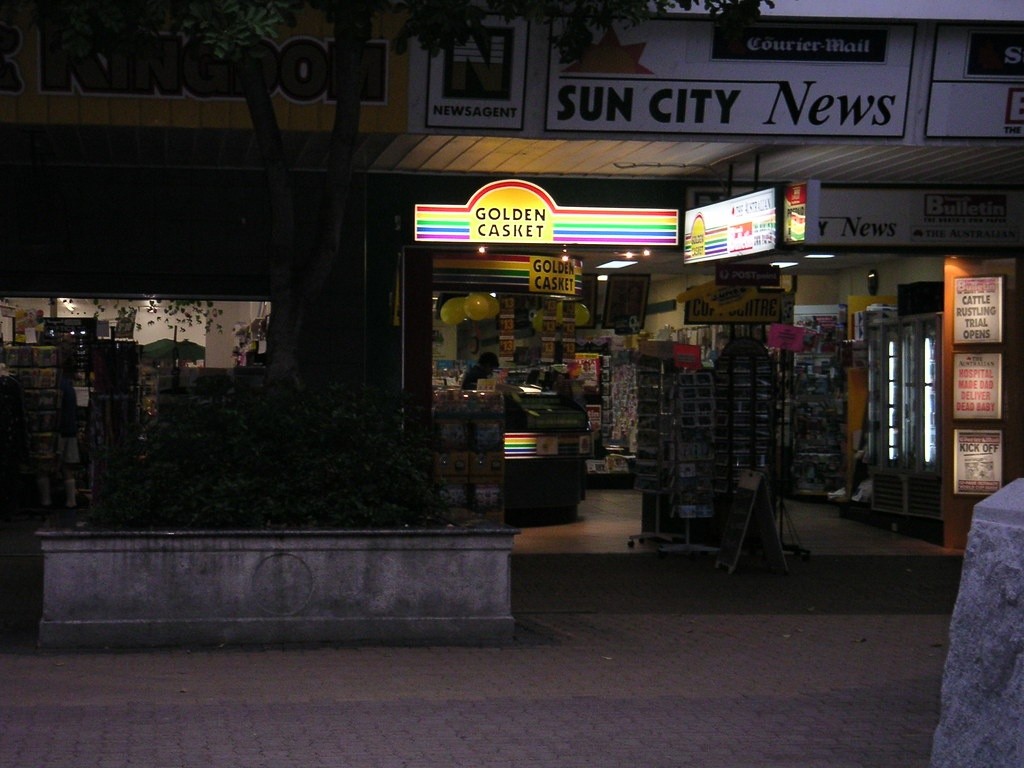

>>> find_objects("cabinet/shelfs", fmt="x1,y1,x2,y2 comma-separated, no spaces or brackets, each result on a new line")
0,304,173,520
569,314,851,558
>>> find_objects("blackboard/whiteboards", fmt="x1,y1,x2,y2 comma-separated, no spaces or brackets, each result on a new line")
715,468,765,574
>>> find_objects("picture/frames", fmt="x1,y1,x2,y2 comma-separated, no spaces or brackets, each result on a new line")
565,277,598,330
601,273,653,330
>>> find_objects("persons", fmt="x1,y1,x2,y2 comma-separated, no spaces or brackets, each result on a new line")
460,351,501,391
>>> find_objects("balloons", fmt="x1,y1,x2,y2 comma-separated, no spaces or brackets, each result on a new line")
439,292,590,333
